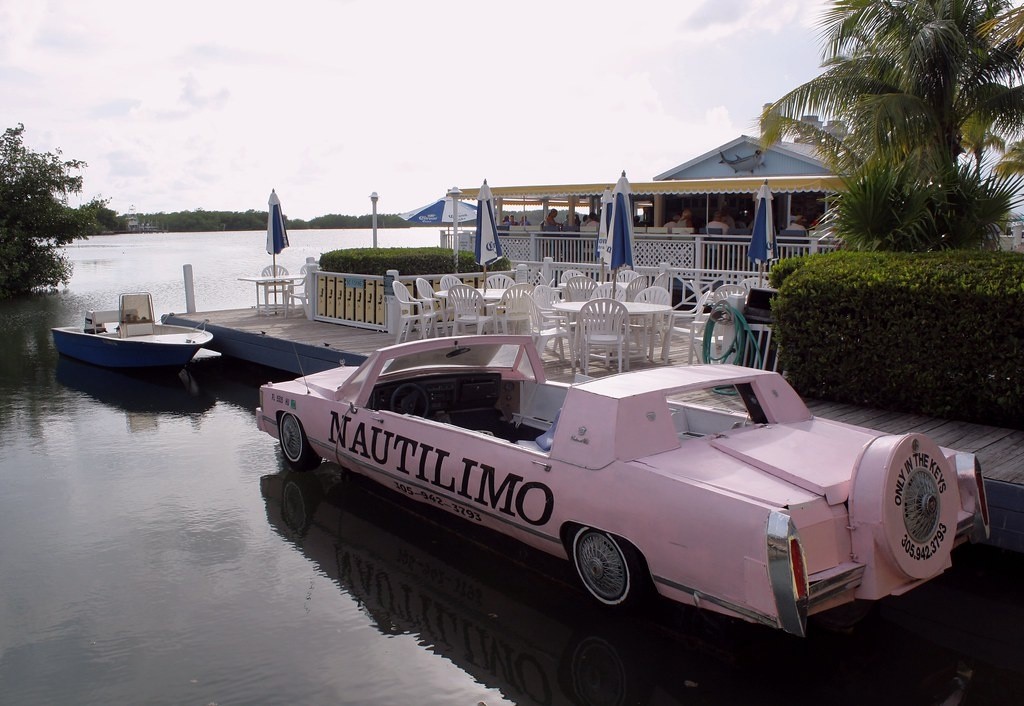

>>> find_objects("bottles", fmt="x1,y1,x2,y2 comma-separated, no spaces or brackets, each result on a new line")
185,339,195,343
559,224,562,231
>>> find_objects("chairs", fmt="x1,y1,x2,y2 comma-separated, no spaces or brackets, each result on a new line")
415,278,455,339
256,265,295,317
479,268,773,376
494,224,845,275
278,272,309,321
392,281,440,345
299,263,321,276
516,407,563,453
438,274,467,335
447,284,493,335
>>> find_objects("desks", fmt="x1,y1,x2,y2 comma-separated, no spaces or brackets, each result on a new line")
237,273,308,320
432,287,534,335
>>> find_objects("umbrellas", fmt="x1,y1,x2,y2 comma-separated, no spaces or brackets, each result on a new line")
397,197,499,223
266,191,288,314
475,184,502,295
603,175,634,306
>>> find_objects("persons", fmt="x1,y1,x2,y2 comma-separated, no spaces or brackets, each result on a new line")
502,215,511,225
510,214,518,225
541,207,823,238
520,216,529,225
595,189,615,285
748,185,779,289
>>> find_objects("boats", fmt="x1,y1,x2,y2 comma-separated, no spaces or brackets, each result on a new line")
253,334,993,641
53,292,214,368
